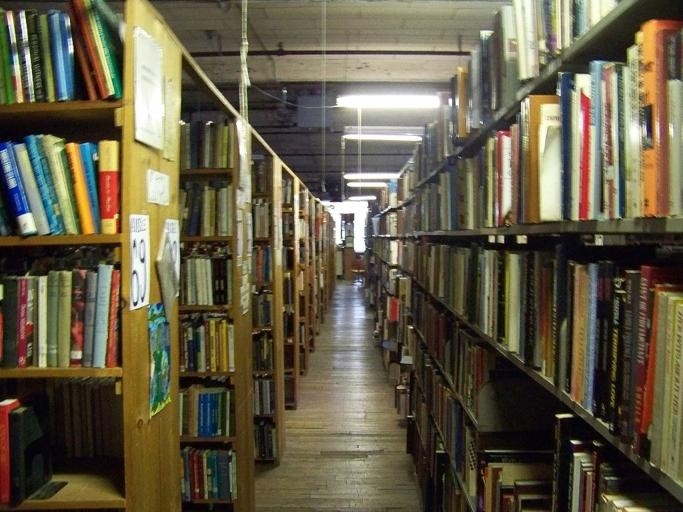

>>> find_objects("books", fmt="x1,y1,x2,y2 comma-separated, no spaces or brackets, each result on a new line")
345,236,354,247
362,0,682,512
180,118,237,512
249,161,333,458
0,0,122,512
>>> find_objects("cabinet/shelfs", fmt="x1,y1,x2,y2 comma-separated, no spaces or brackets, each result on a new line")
252,155,285,466
283,175,300,408
1,2,183,511
299,189,336,373
363,0,682,512
180,119,256,511
